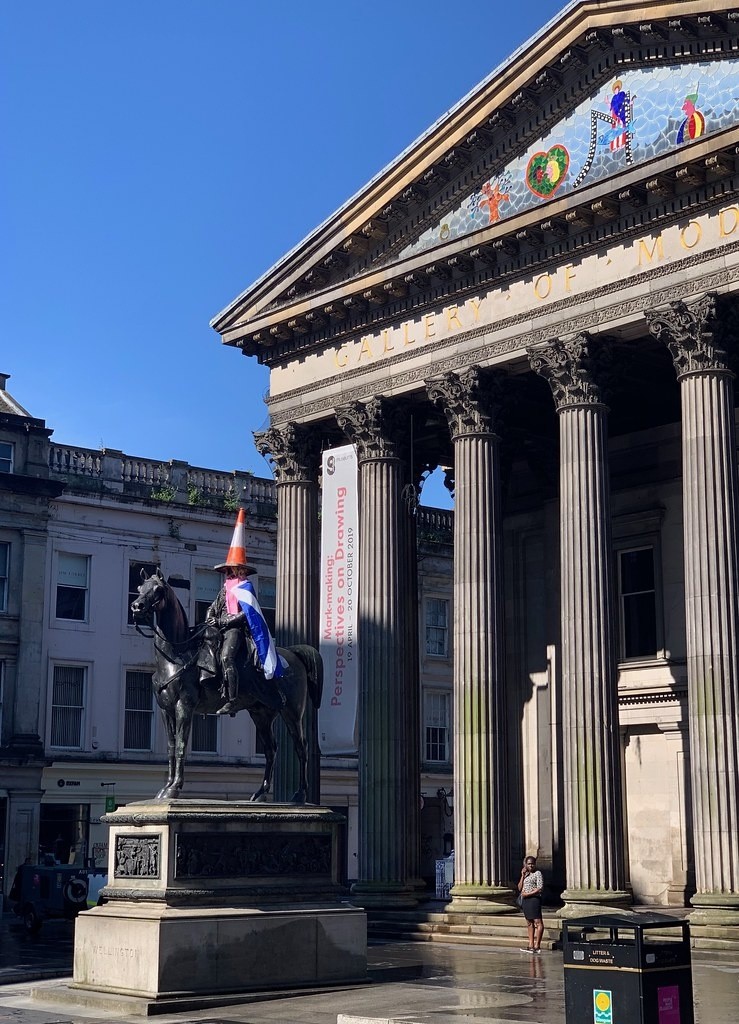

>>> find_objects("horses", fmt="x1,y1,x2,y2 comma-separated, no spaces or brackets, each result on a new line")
130,567,325,806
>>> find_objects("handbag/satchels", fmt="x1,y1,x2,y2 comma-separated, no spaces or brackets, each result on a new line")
515,892,524,908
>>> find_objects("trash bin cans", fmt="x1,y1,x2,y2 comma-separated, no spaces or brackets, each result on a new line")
562,911,695,1024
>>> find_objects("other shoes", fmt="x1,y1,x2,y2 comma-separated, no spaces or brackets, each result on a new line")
519,947,542,955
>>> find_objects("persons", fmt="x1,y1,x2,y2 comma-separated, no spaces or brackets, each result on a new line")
206,568,254,716
519,856,543,952
